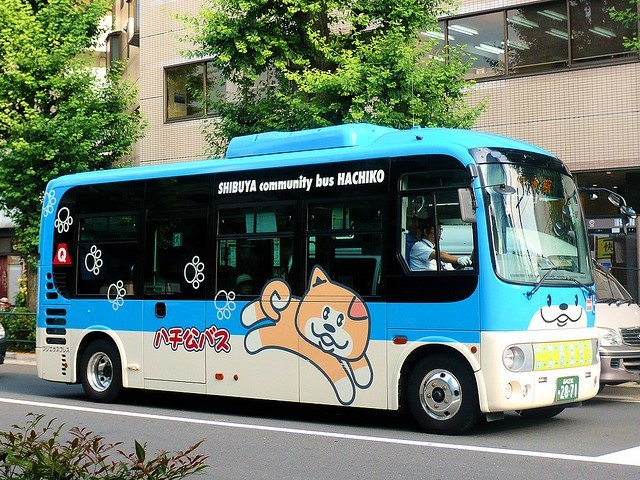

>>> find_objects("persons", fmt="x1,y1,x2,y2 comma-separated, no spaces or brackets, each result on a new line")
409,216,472,271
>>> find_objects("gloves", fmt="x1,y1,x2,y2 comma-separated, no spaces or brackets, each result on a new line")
457,257,472,266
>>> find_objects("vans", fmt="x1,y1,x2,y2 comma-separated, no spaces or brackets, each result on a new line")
291,223,638,396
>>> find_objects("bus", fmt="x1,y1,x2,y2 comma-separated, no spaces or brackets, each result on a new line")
575,185,635,273
36,124,626,434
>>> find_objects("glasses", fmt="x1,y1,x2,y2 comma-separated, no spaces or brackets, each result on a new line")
440,228,443,234
